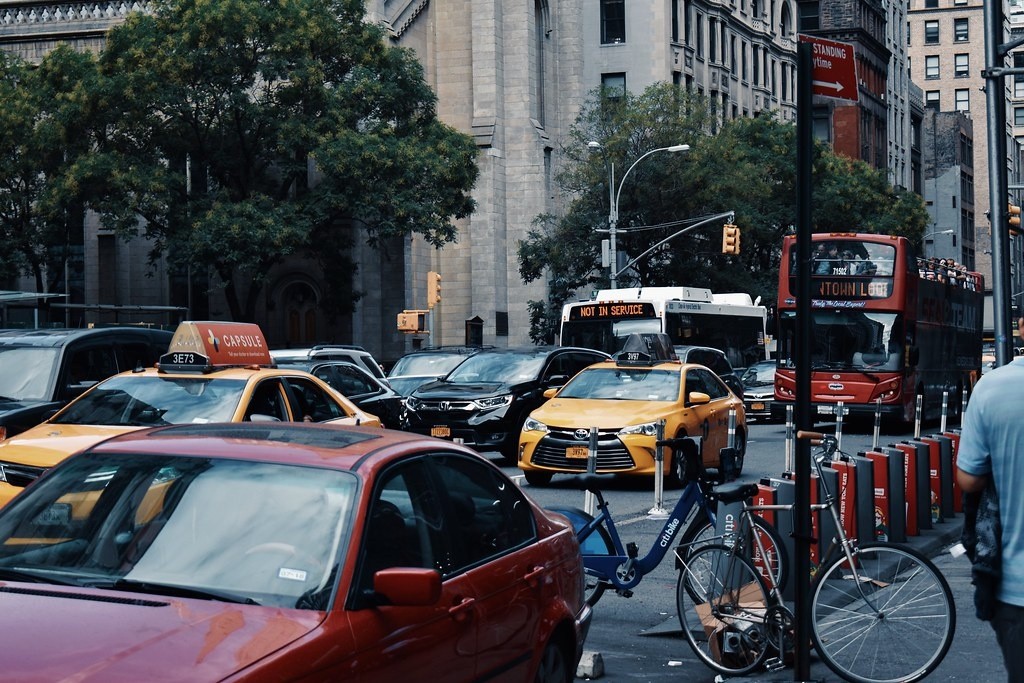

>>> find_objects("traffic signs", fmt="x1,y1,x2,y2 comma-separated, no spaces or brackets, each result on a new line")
798,33,858,104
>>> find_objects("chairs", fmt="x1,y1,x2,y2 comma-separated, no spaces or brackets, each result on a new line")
363,500,409,573
275,387,307,422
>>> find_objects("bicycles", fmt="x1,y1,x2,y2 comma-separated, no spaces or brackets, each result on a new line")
544,438,789,609
672,431,956,683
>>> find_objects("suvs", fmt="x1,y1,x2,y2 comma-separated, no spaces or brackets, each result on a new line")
384,344,493,399
0,328,176,442
268,343,390,396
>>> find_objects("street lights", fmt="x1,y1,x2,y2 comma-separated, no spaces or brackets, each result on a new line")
589,139,690,287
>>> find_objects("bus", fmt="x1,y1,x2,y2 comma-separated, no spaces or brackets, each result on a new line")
557,285,771,370
775,233,984,430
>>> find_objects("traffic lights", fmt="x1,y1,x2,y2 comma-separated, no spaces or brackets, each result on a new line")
427,271,441,308
722,227,737,253
397,312,425,332
1008,204,1021,237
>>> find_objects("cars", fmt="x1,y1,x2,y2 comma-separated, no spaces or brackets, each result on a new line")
740,359,776,422
0,321,381,562
517,333,748,492
278,361,402,430
402,344,611,463
670,344,744,400
0,424,594,682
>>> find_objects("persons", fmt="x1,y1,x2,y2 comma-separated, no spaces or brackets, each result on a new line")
867,326,901,354
955,316,1023,683
790,242,977,291
